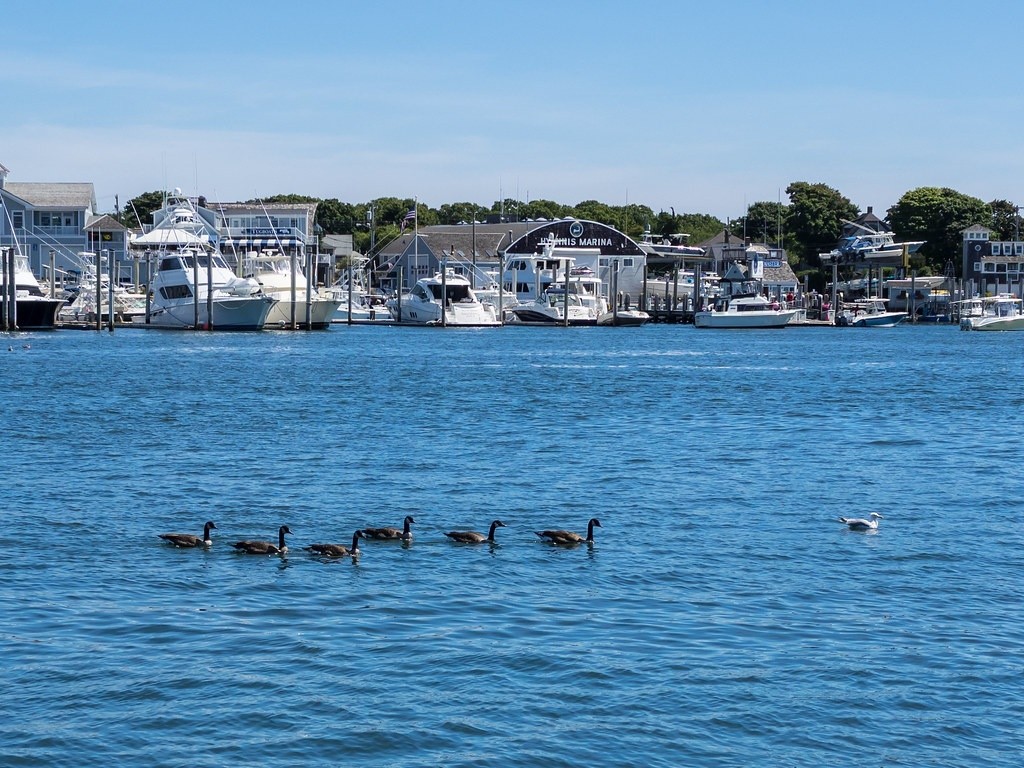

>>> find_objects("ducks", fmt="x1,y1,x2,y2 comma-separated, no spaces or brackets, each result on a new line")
229,526,294,555
157,521,217,547
443,519,602,545
839,512,883,529
299,516,415,558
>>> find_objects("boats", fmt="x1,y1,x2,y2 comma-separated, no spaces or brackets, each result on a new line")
388,269,500,326
949,294,1024,331
512,265,608,326
232,248,346,330
638,233,705,257
693,278,803,328
818,230,927,261
594,310,652,326
927,291,951,301
0,192,68,330
129,189,280,330
58,247,153,323
640,257,723,299
322,286,392,322
840,297,910,327
473,232,576,323
824,278,947,291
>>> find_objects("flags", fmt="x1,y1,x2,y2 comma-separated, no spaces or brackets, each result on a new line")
401,202,416,232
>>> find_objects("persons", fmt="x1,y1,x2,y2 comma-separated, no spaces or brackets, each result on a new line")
787,292,793,301
984,291,992,310
772,299,780,310
